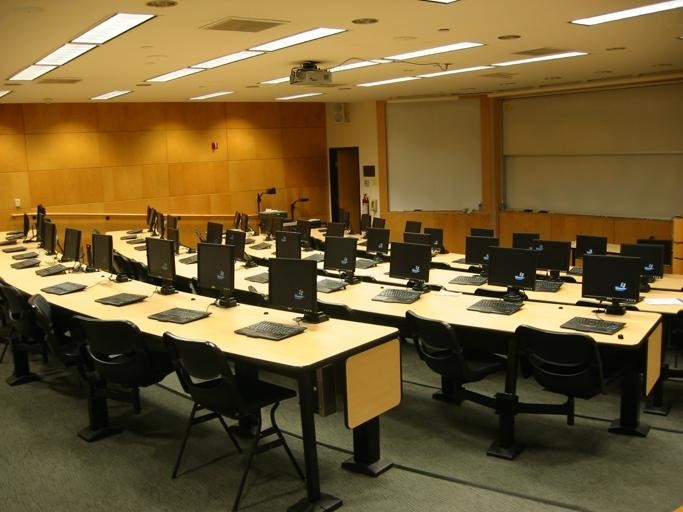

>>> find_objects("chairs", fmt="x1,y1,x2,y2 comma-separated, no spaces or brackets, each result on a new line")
406,280,445,292
27,294,80,361
0,283,49,364
369,253,391,264
308,237,322,251
85,244,93,267
513,324,631,401
162,332,305,512
535,273,577,284
353,249,370,258
340,272,377,284
574,301,639,311
112,252,130,275
405,309,509,385
473,288,529,302
70,315,176,388
430,261,451,270
467,266,482,273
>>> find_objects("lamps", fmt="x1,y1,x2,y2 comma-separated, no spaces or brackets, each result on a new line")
290,197,309,222
257,187,276,212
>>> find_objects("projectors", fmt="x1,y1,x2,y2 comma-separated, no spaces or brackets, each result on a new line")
289,69,333,86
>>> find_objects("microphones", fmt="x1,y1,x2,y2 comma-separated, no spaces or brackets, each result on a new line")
291,193,310,206
259,182,277,197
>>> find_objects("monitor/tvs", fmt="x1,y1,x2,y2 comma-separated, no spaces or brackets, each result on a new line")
91,232,130,283
268,257,329,324
37,212,51,249
425,228,449,254
341,210,354,235
275,231,302,259
389,241,430,293
403,232,433,261
372,217,387,230
296,220,314,250
167,214,178,229
582,253,641,316
197,242,237,308
405,221,421,234
227,228,258,268
513,232,541,249
38,203,44,214
324,237,359,285
147,206,155,232
361,213,373,231
146,235,176,295
241,212,248,232
152,208,158,237
487,245,537,304
234,210,241,227
637,239,676,267
575,235,607,257
366,228,391,263
327,223,345,238
272,217,285,237
534,238,572,282
63,227,96,272
22,211,36,243
470,228,496,237
465,236,500,275
206,222,224,244
620,243,666,293
44,220,71,262
157,213,165,239
167,228,180,255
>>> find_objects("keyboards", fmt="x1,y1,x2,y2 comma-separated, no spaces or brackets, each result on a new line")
13,252,40,260
358,240,368,246
41,282,88,296
128,227,143,233
356,241,367,246
355,255,379,269
249,242,271,250
525,280,563,292
2,246,29,253
372,288,423,303
95,292,148,307
301,253,324,262
449,276,489,286
7,232,25,241
596,295,646,304
467,299,525,315
452,258,465,265
319,227,327,233
120,235,138,241
566,266,584,276
135,245,148,251
362,234,368,239
11,258,42,271
180,254,199,265
147,307,212,324
245,237,254,244
318,279,347,293
235,321,306,340
1,239,16,245
34,264,68,276
7,230,23,235
244,273,269,283
560,316,627,334
127,238,146,244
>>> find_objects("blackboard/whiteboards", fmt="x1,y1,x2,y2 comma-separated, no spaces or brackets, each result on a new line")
501,82,683,221
384,96,483,212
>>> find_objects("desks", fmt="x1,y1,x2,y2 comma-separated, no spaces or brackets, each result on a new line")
103,227,671,438
0,230,402,511
309,227,683,292
221,233,683,315
